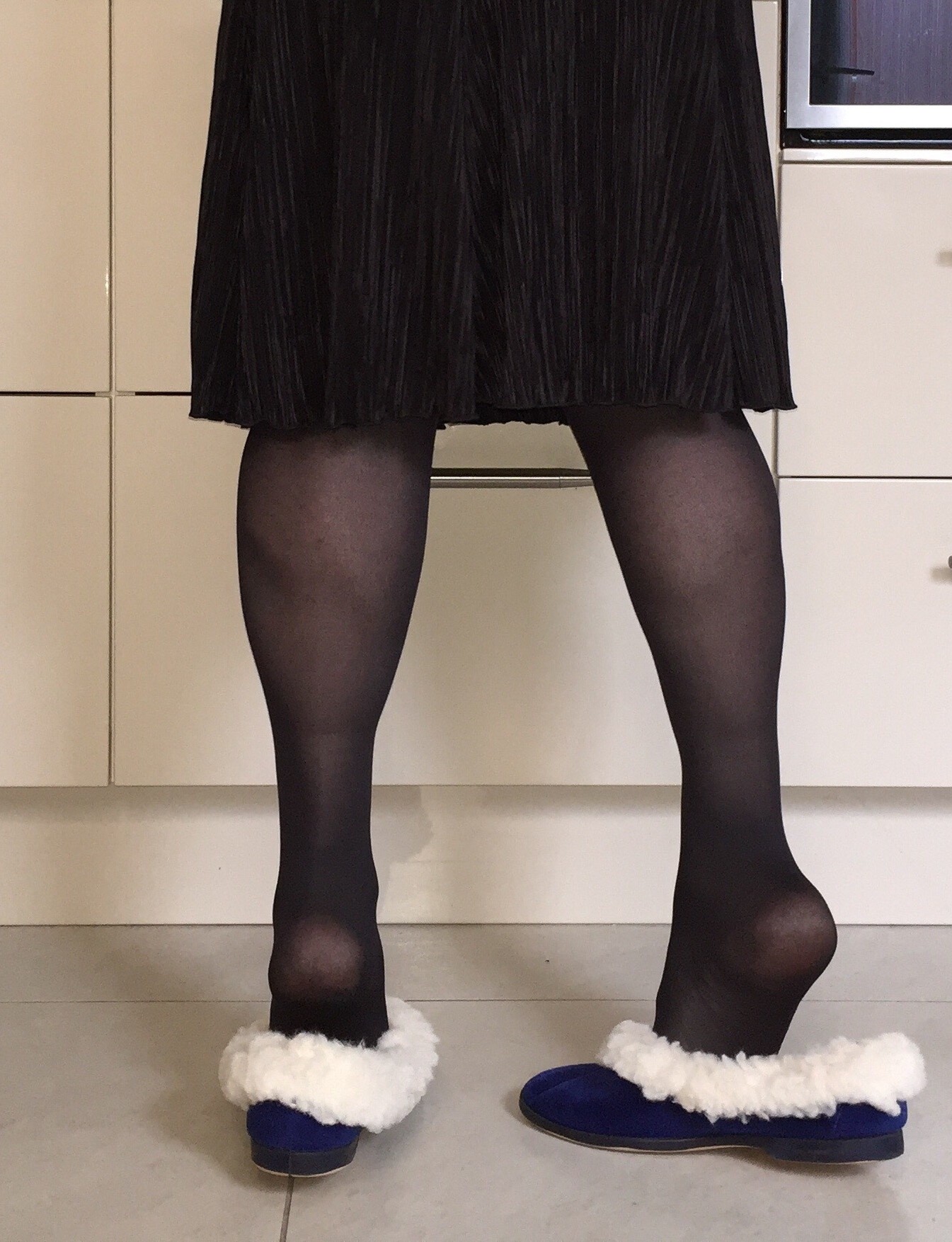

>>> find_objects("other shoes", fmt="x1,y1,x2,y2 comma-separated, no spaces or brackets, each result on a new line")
520,1019,926,1166
220,993,440,1179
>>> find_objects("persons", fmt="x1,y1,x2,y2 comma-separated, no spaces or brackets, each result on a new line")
187,0,927,1177
